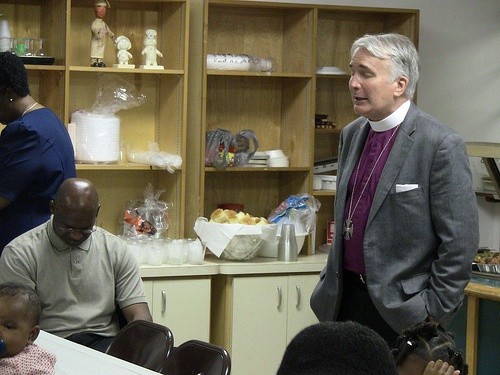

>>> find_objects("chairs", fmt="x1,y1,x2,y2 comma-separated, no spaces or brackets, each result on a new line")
159,340,231,375
105,320,174,372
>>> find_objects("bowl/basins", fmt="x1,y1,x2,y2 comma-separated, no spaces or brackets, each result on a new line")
258,233,309,258
218,234,271,261
472,262,500,274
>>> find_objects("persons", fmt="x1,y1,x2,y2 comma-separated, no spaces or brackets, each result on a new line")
392,319,468,375
114,35,133,64
310,35,480,354
0,178,153,354
0,281,56,375
277,320,399,375
141,29,163,66
91,0,115,67
0,51,77,257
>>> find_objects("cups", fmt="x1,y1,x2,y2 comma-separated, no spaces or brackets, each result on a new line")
0,36,47,56
118,235,206,266
277,224,298,262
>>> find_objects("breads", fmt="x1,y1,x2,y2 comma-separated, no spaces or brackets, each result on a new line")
474,251,500,264
209,208,268,224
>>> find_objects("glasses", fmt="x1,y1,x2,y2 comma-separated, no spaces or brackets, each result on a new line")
53,207,97,234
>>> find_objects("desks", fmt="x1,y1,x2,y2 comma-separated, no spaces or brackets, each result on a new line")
33,329,163,375
464,283,500,375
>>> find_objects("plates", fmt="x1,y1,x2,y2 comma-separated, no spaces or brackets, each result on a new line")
70,112,119,165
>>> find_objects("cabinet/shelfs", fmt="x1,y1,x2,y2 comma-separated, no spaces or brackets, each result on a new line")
0,0,420,375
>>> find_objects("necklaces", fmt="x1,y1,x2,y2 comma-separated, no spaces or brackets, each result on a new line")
22,101,38,116
342,124,399,241
